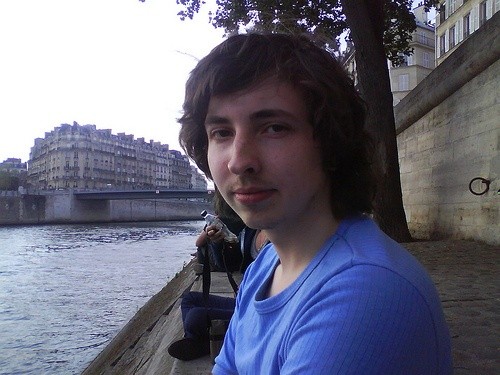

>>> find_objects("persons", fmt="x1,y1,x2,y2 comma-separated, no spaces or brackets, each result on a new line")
168,209,273,360
175,32,452,375
188,181,193,189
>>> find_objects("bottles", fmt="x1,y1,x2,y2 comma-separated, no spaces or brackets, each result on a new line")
201,210,239,248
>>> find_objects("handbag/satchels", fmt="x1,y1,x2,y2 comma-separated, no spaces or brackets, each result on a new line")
209,319,231,366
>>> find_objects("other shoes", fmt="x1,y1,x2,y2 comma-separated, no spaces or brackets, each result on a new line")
168,338,211,361
